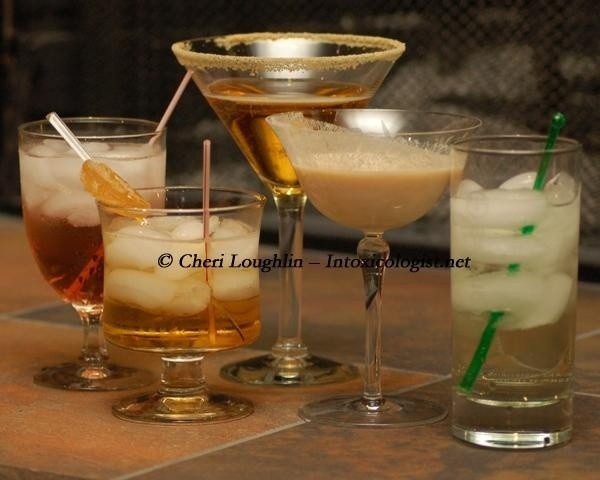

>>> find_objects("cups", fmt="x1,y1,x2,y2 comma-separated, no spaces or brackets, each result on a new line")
447,133,584,452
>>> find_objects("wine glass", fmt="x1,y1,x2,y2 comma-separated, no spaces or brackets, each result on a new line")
264,107,483,430
17,114,170,392
169,26,409,388
95,185,267,426
